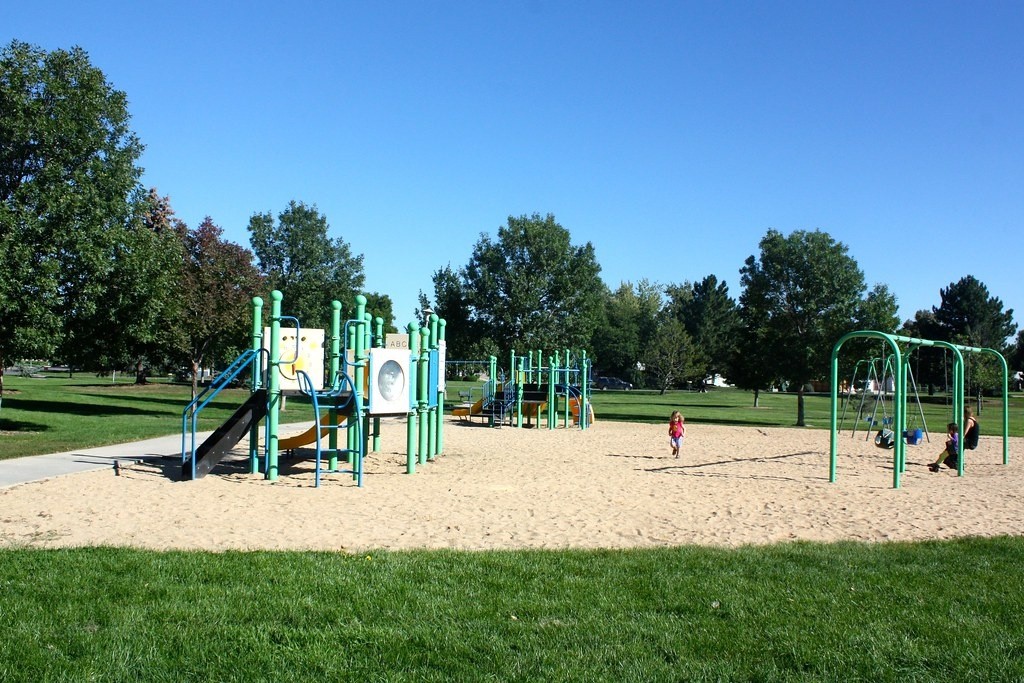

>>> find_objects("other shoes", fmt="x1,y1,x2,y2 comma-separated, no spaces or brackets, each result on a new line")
929,467,939,472
927,463,939,468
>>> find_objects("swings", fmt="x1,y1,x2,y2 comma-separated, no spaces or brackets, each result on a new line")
866,341,971,471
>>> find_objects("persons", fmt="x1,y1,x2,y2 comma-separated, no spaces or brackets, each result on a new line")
668,410,685,458
927,423,959,473
963,406,979,450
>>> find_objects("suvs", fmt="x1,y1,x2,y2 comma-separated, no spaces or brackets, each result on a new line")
596,376,633,391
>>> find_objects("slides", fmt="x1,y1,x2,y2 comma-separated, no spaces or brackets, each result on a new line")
513,402,547,417
452,382,506,416
278,412,348,450
